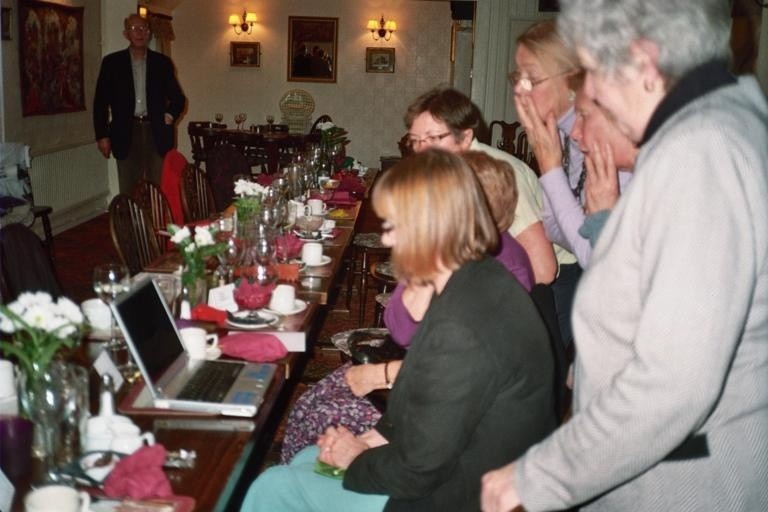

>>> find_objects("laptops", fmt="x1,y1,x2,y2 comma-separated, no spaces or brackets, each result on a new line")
110,274,277,418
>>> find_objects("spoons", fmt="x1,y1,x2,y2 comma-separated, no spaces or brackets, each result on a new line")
58,451,114,484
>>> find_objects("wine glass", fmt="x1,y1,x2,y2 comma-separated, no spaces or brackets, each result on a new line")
266,115,274,132
214,112,223,129
235,112,247,132
212,132,346,287
93,261,130,352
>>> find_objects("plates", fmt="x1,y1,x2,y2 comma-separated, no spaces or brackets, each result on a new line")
226,309,277,330
266,299,306,314
191,347,221,362
91,499,120,511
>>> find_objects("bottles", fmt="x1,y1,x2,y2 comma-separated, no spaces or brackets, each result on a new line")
95,376,117,443
209,120,213,128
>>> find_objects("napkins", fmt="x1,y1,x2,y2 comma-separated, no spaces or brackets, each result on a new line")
181,283,239,319
227,331,306,353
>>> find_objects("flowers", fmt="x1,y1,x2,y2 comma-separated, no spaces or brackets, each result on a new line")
0,289,88,412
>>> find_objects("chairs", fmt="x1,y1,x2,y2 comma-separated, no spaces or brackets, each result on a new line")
0,141,59,271
489,118,539,174
104,113,334,281
0,223,66,306
330,156,398,363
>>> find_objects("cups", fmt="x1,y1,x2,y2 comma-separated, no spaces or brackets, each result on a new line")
24,486,90,511
0,359,17,399
83,298,110,330
269,284,296,311
0,415,35,507
79,454,122,481
178,326,218,357
84,418,143,455
145,273,176,315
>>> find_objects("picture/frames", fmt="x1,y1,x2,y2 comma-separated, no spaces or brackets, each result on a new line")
288,16,339,83
0,8,14,41
365,47,396,74
229,41,260,68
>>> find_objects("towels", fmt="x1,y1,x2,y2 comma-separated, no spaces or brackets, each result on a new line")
101,441,196,510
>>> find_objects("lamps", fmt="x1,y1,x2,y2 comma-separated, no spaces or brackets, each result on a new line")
367,16,398,41
228,9,257,36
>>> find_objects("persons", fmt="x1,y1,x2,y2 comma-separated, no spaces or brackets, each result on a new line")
93,13,186,197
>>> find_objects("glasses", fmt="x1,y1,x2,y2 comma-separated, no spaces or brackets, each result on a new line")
407,132,451,149
128,25,146,32
508,72,570,91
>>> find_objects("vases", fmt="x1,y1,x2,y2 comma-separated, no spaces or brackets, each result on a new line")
14,369,75,489
180,266,208,315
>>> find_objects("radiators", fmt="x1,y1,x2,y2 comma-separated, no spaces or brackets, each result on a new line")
21,140,111,221
